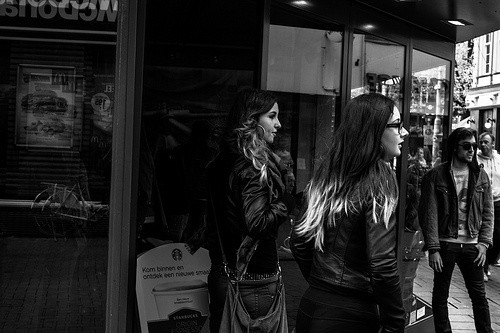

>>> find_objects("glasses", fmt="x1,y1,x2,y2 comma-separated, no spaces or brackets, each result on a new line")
457,144,477,150
386,122,403,129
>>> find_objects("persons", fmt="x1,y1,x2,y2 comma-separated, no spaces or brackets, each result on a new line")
204,89,296,333
289,93,410,333
417,128,495,333
475,131,500,281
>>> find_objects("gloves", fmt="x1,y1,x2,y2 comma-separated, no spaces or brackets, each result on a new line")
282,186,303,215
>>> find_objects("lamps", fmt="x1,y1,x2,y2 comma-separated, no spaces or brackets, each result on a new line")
466,119,475,128
485,118,495,129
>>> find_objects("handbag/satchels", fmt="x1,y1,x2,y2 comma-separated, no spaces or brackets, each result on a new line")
218,272,288,333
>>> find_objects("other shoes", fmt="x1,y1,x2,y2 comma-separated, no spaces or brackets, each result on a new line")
483,264,491,282
